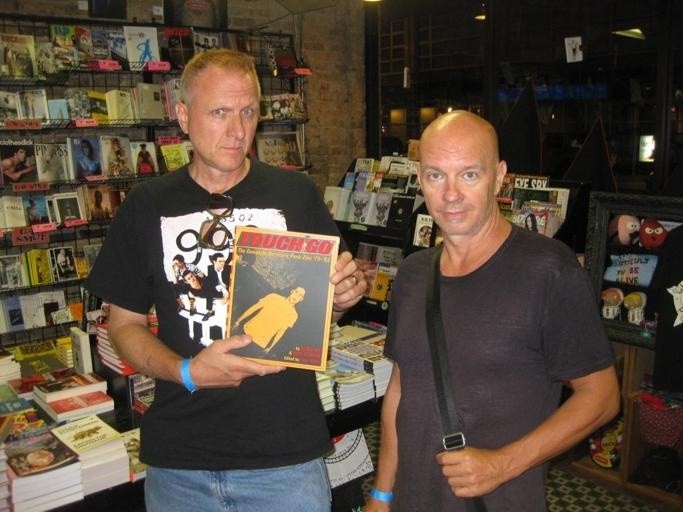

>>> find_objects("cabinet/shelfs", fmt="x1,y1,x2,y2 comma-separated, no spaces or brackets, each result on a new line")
2,14,397,510
570,339,680,507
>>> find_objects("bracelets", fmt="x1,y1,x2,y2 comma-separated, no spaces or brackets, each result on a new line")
370,490,393,503
180,358,197,393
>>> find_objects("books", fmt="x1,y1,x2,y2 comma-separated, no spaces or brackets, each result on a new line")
197,32,311,170
321,155,444,307
1,20,195,233
226,225,340,372
316,322,395,413
495,174,569,245
2,242,160,511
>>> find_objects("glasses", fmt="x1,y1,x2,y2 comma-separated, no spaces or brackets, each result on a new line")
198,193,234,250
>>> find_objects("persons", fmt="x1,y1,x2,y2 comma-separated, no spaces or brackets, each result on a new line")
359,110,624,512
82,48,367,511
170,253,231,311
